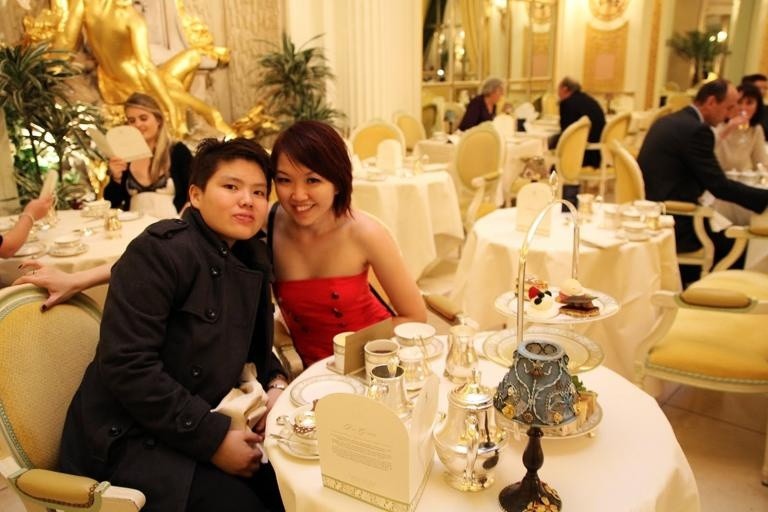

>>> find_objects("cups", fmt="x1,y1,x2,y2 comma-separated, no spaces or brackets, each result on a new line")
394,322,436,346
576,194,593,214
364,339,399,385
56,236,82,248
601,199,661,233
332,331,354,370
85,199,112,214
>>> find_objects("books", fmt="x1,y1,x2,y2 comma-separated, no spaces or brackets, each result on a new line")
87,125,154,163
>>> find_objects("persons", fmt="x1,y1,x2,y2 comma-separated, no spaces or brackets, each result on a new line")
56,137,287,511
11,118,428,373
1,183,50,259
458,77,504,132
636,73,768,292
547,77,606,212
103,93,195,214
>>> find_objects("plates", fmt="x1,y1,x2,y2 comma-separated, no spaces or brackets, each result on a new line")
390,337,444,362
496,402,603,440
11,241,46,258
496,288,619,324
290,374,365,408
119,210,145,222
482,326,603,374
277,426,322,459
289,405,316,426
48,244,90,256
619,235,648,241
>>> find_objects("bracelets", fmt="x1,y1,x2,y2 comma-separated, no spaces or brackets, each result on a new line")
269,383,285,390
19,213,34,224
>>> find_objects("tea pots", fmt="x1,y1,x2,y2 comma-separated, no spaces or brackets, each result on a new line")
445,325,481,385
368,364,413,421
432,368,508,492
